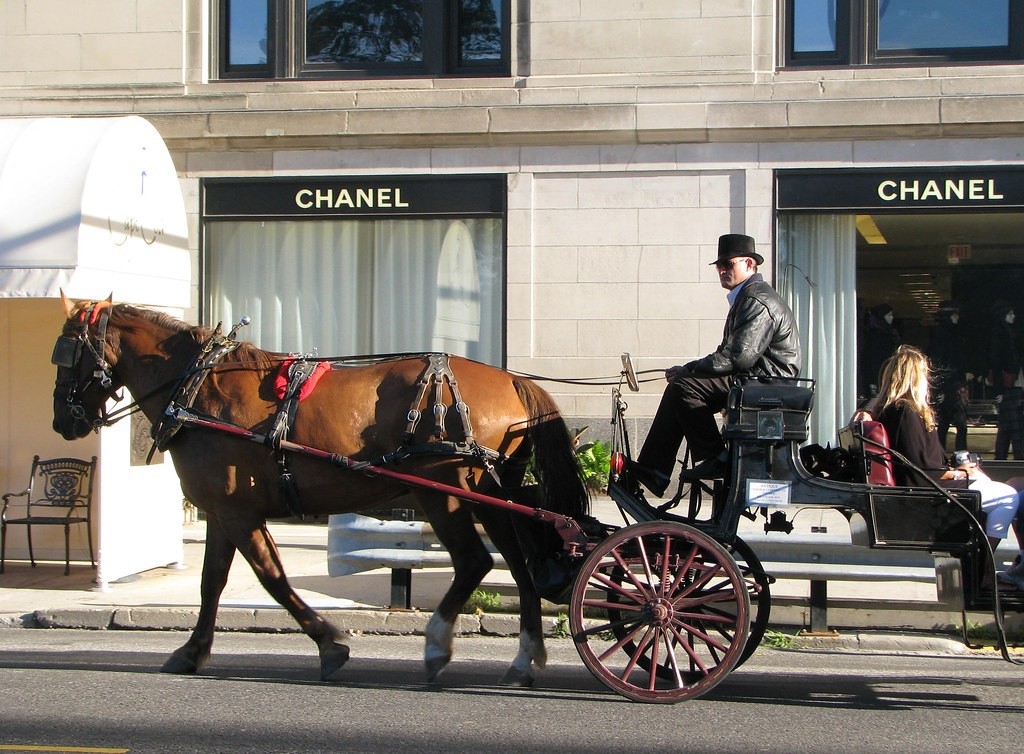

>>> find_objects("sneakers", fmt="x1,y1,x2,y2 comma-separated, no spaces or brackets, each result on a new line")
994,557,1017,585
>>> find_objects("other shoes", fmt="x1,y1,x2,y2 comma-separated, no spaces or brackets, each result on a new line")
679,451,734,480
620,452,670,495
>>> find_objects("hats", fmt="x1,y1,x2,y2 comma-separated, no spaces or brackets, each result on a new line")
933,300,961,315
709,234,764,266
990,297,1015,314
869,303,893,317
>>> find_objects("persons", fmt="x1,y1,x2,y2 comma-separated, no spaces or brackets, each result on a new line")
860,301,1024,460
610,234,802,497
872,344,1024,588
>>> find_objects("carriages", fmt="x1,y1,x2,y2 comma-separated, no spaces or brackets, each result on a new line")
47,286,1024,707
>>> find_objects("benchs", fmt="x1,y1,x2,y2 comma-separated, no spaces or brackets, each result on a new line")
727,376,817,440
837,409,896,487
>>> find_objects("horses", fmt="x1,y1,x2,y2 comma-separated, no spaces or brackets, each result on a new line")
53,288,597,687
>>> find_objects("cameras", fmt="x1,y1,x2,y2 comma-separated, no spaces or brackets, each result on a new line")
952,450,979,469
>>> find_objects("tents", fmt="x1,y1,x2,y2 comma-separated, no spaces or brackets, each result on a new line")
0,117,190,585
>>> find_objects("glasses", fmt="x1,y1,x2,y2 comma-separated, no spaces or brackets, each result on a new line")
716,259,750,270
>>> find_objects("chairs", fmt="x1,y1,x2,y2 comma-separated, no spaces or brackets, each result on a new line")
0,454,96,576
